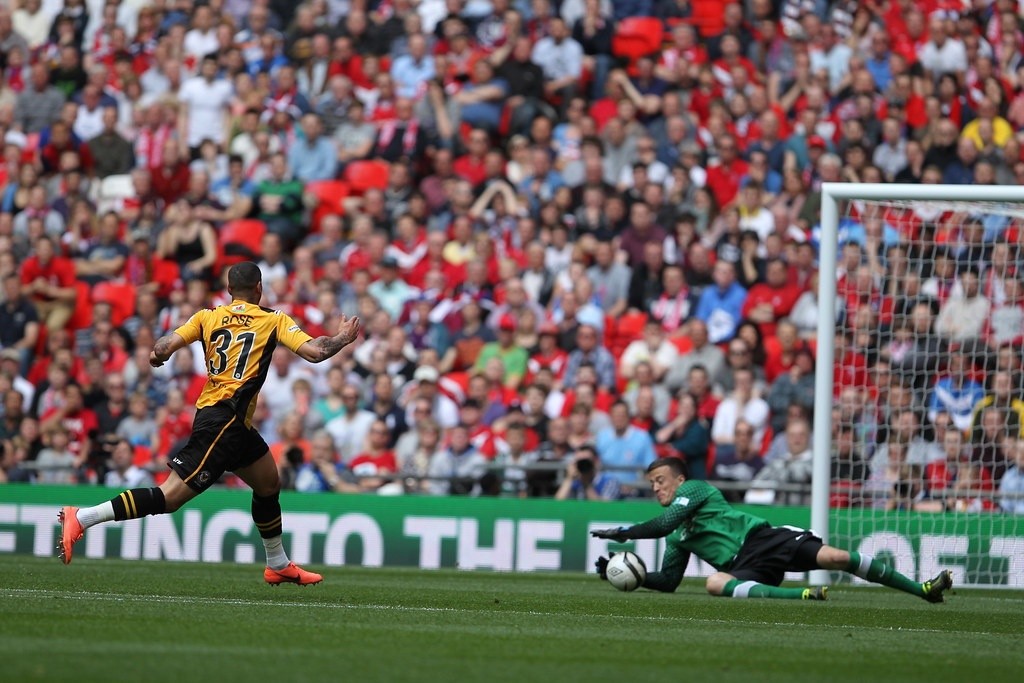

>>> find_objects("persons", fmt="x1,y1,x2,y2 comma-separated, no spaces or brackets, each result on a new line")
589,457,954,605
57,255,360,587
0,1,1024,514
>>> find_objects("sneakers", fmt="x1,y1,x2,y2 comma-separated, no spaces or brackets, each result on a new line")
264,560,323,587
922,568,953,602
802,585,830,600
57,506,84,566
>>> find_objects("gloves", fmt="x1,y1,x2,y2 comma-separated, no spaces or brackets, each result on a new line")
596,552,615,580
591,526,626,543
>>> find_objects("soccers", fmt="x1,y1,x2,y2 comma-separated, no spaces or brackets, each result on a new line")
605,551,647,592
